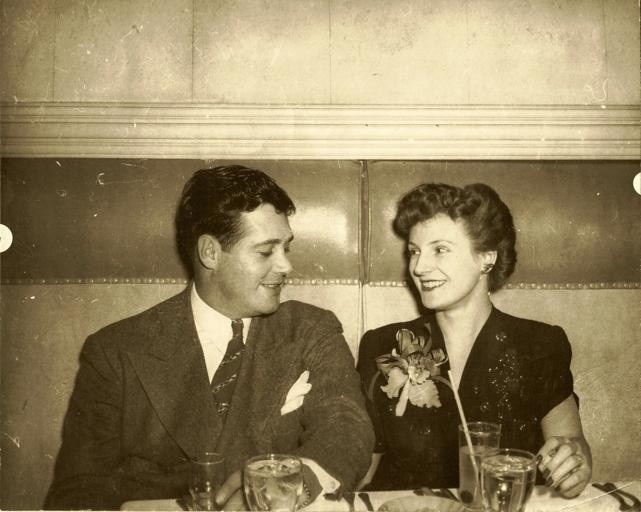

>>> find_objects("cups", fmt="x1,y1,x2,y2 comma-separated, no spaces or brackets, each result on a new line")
478,446,537,512
457,423,502,510
187,453,225,510
242,453,304,511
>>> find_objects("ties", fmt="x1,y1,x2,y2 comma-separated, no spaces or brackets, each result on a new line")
211,319,245,421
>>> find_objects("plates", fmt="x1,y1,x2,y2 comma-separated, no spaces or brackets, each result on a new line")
377,493,466,511
